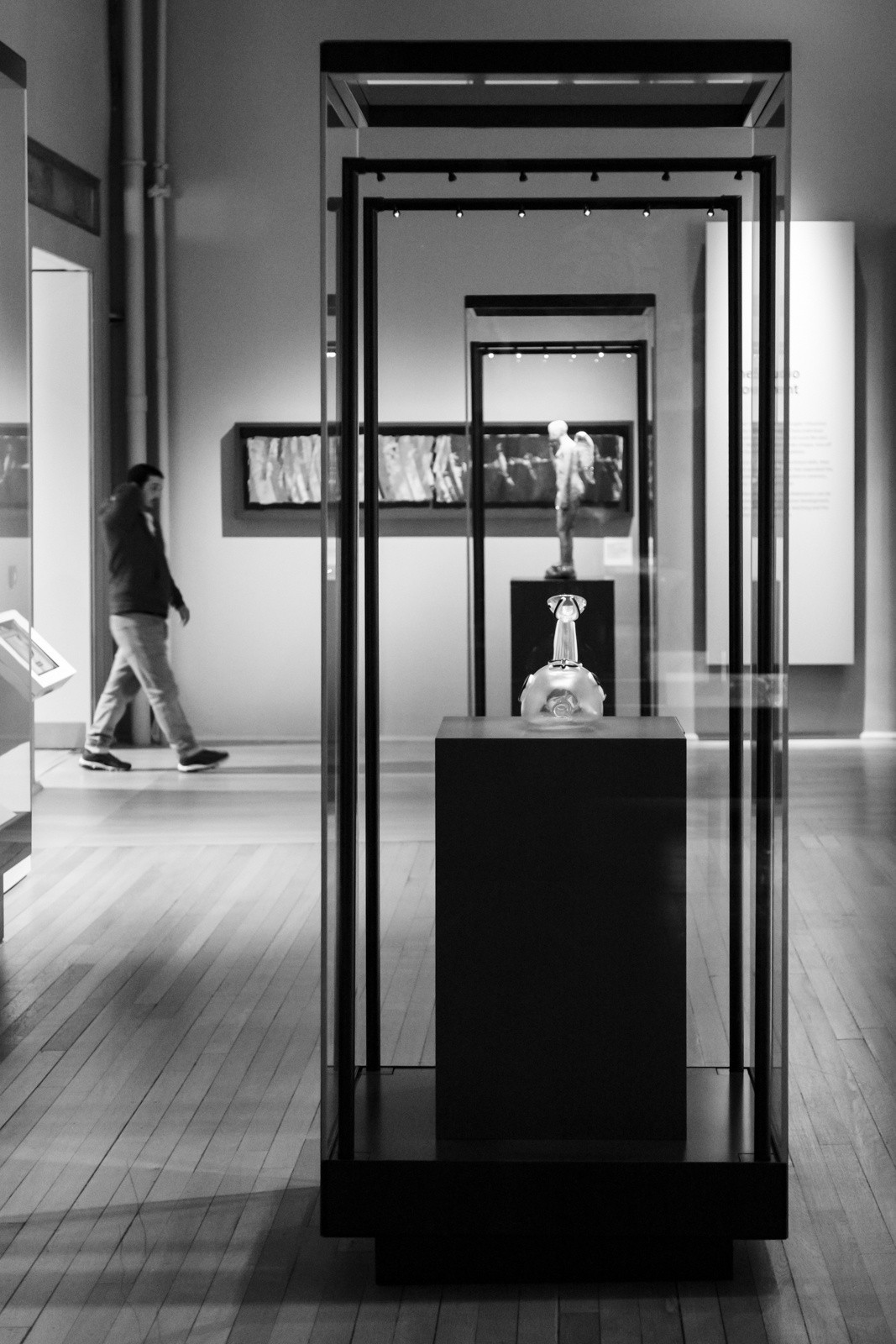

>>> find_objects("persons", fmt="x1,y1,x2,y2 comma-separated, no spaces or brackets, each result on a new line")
545,421,596,581
78,464,230,773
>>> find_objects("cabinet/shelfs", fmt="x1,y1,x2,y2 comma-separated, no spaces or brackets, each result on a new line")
230,419,638,518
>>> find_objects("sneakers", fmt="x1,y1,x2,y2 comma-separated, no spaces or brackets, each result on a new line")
178,750,229,772
80,749,131,772
